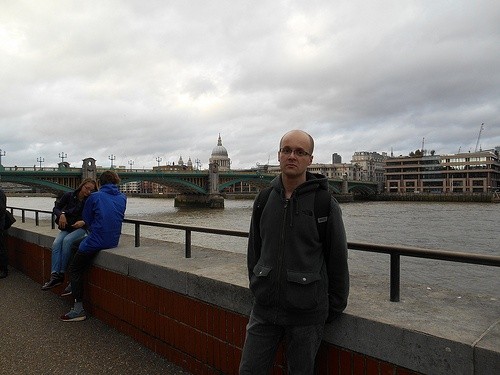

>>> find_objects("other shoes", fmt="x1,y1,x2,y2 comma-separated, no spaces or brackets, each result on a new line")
60,282,72,297
0,268,8,279
41,272,65,291
61,301,87,322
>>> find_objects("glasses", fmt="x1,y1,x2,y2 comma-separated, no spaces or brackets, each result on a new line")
280,147,311,157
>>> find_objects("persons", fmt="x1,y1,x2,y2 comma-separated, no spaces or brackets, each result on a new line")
0,188,10,279
61,170,127,321
40,177,98,291
239,131,349,375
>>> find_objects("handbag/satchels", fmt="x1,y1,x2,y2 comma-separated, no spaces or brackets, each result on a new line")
0,210,16,232
